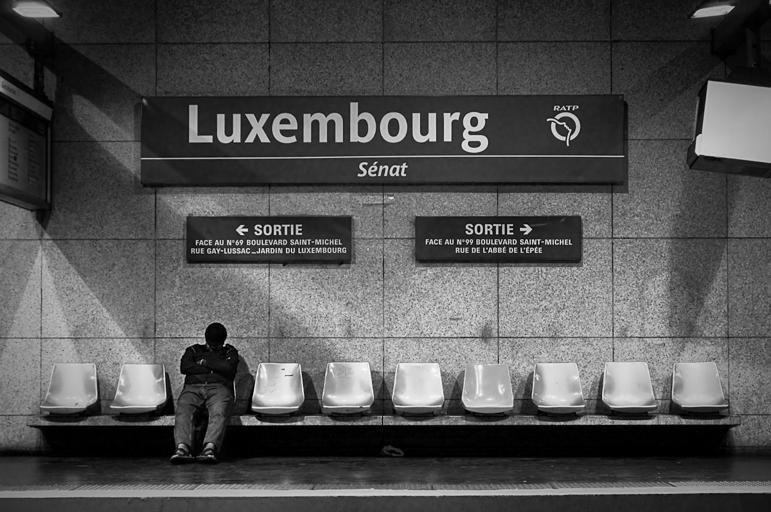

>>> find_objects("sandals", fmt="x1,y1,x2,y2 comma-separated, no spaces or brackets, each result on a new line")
169,446,218,462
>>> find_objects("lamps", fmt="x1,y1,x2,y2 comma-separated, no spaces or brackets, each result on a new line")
688,0,736,19
11,0,63,18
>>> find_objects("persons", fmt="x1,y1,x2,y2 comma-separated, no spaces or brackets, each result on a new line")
170,321,239,464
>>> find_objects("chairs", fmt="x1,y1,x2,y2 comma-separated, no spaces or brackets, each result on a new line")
321,361,375,413
672,362,728,408
251,362,305,414
462,363,514,414
40,362,98,414
391,362,443,412
233,380,237,405
600,362,658,413
109,363,166,413
532,362,585,413
